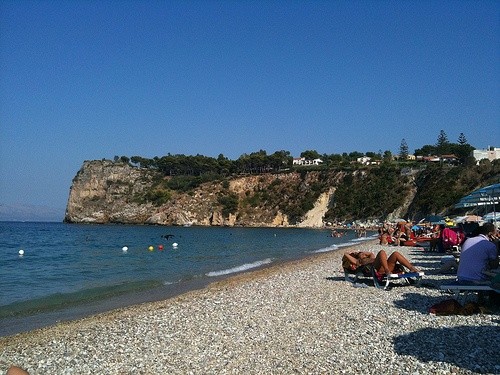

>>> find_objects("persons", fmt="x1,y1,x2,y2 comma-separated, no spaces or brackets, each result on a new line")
323,217,499,302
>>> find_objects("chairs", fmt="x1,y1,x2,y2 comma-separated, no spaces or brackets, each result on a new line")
440,284,500,306
376,222,461,254
342,257,424,290
